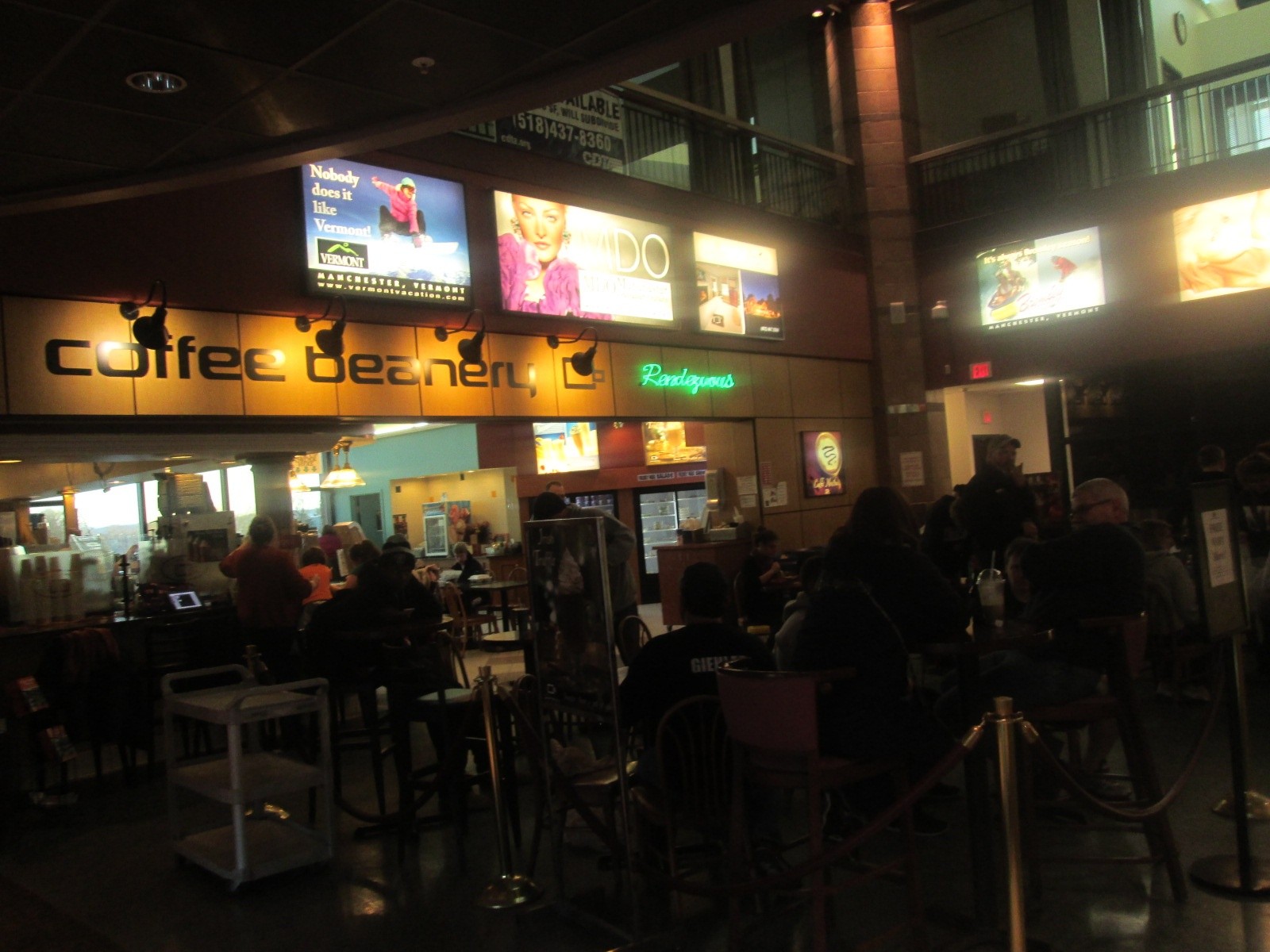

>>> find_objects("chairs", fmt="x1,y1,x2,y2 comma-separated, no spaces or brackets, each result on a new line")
446,581,499,657
631,695,731,905
28,617,212,794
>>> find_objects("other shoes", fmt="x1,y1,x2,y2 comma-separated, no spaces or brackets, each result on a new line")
926,782,958,798
913,817,946,835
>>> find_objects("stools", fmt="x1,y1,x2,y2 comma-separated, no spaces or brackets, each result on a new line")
717,656,930,952
1024,611,1188,904
398,685,522,874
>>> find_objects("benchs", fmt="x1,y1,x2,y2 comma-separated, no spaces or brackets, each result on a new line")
481,604,532,646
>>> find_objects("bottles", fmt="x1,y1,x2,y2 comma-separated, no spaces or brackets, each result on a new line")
36,523,48,546
115,571,124,603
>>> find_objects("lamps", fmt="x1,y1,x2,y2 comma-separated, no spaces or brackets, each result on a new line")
296,295,347,357
435,309,487,364
548,326,598,375
121,279,169,349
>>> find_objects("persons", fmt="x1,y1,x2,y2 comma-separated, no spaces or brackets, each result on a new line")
497,192,616,321
221,432,1270,952
373,175,429,247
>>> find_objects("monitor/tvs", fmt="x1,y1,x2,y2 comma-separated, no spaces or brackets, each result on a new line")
296,158,475,311
1169,184,1270,306
490,189,684,332
974,225,1109,338
690,231,788,341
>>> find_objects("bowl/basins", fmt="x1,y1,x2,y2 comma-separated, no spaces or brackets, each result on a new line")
467,574,492,583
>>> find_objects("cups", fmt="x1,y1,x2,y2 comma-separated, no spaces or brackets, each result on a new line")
746,625,770,644
976,568,1006,628
20,553,84,629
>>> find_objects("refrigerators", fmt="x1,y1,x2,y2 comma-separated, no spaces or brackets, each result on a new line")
528,489,620,522
422,500,471,557
631,481,708,605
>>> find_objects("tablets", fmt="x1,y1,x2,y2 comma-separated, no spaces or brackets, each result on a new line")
167,591,204,612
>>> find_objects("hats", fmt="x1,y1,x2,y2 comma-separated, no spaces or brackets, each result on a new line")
378,534,415,562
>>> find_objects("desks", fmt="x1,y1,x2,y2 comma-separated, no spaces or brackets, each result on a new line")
930,619,1055,952
652,539,748,633
470,581,533,652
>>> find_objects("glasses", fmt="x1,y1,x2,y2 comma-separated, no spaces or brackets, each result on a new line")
1070,500,1110,517
